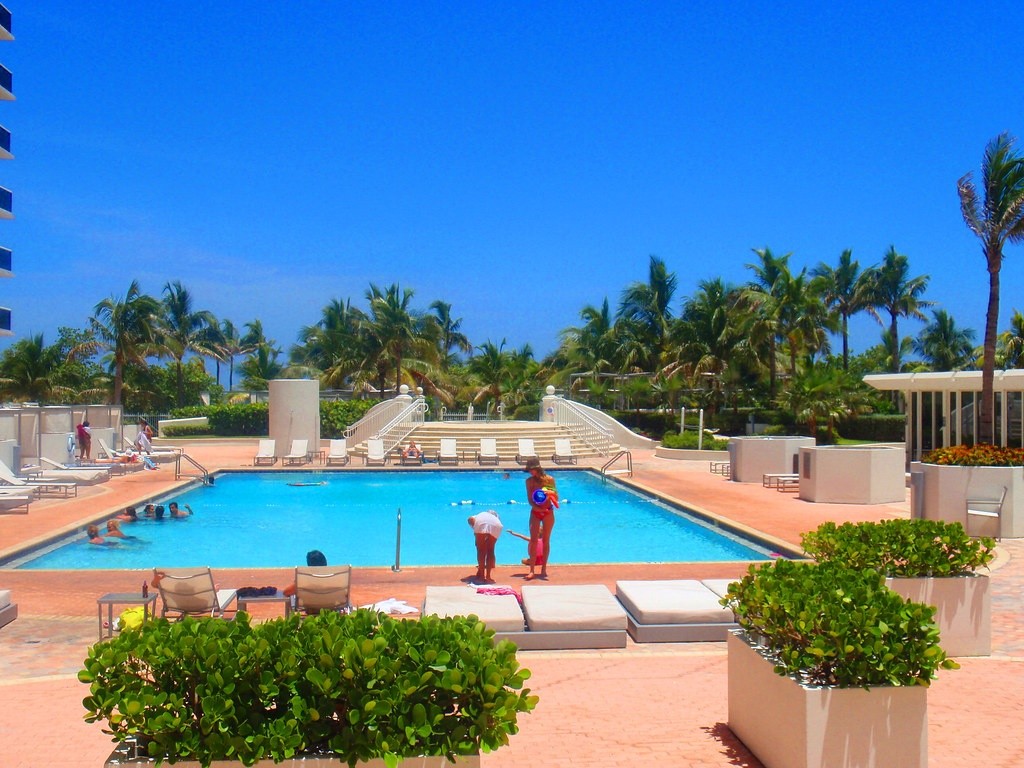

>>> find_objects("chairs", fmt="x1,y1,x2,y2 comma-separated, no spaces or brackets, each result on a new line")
710,461,730,476
282,439,309,466
291,565,351,616
552,439,578,465
436,439,459,466
152,568,238,621
965,487,1007,543
365,440,386,466
516,439,539,464
326,438,349,465
253,439,276,466
475,438,499,467
0,438,185,514
763,474,799,492
403,444,423,465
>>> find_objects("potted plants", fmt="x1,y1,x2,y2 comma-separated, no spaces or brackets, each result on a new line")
718,518,997,768
78,605,539,768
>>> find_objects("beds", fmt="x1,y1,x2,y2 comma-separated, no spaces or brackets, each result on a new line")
121,451,180,463
423,578,747,650
0,589,18,629
30,468,109,486
0,486,33,509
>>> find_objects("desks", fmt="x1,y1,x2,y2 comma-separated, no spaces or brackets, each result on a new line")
98,592,159,642
350,450,364,464
237,590,292,620
307,450,325,465
387,450,404,464
97,451,116,459
19,465,41,472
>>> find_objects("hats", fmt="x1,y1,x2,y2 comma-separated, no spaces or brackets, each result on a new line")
522,457,541,472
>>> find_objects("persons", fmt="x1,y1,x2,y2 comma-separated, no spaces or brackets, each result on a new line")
143,504,165,519
134,418,154,455
524,456,559,579
105,519,124,537
400,440,422,459
151,571,221,611
506,526,544,566
86,525,105,545
77,421,95,459
169,502,194,518
283,550,350,606
79,454,132,464
116,507,138,522
467,510,503,583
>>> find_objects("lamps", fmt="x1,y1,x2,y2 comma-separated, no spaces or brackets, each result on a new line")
950,370,959,382
910,372,917,383
414,387,423,394
997,369,1007,380
547,385,555,395
400,384,409,395
